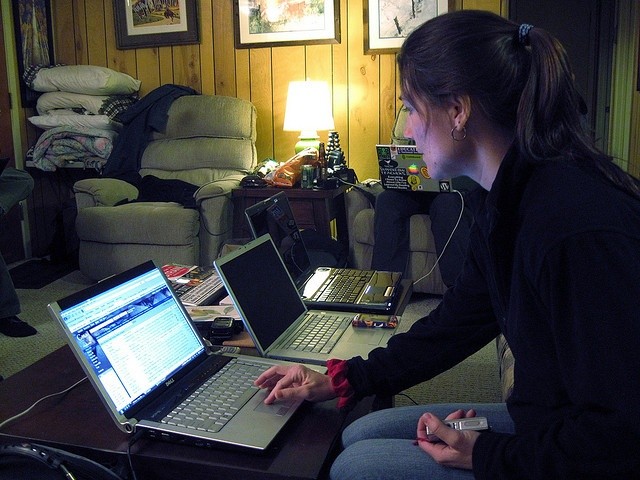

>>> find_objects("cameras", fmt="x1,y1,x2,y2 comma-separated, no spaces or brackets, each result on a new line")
327,164,356,185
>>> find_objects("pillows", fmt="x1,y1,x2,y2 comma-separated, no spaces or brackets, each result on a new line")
28,65,142,96
26,115,124,131
36,91,139,116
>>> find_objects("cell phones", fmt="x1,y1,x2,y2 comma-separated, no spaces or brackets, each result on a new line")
211,316,235,334
425,416,488,443
352,314,398,329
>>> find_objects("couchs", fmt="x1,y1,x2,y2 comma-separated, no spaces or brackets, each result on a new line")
343,104,449,296
73,96,258,281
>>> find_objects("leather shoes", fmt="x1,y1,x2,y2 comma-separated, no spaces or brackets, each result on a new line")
1,316,37,337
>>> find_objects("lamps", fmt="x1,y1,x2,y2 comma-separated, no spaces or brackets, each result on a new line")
282,77,336,155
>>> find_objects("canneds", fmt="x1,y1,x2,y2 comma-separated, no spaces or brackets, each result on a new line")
300,164,314,189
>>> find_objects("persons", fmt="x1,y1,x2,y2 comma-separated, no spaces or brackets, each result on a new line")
252,10,640,480
21,10,33,69
370,139,481,289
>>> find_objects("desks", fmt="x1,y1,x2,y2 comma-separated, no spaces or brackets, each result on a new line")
231,183,353,269
0,278,413,480
24,167,100,257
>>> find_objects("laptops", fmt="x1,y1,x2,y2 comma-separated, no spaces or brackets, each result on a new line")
212,232,402,366
46,259,329,456
244,191,403,313
376,144,453,194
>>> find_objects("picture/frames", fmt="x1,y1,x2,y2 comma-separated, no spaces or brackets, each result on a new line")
362,0,463,55
233,0,341,49
12,0,54,108
112,0,201,50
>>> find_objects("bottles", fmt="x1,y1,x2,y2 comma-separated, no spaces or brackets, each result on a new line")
318,143,328,183
308,151,321,186
